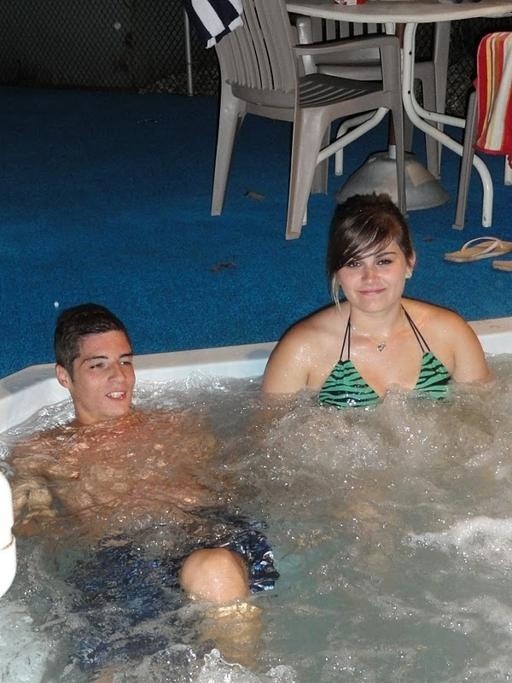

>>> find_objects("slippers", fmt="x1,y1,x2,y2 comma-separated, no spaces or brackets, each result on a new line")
444,236,512,262
492,259,511,271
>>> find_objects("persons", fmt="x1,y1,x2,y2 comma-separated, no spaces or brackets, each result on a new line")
256,190,494,420
10,301,277,683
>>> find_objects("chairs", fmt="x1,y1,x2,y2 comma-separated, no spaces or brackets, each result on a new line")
297,16,450,181
189,0,409,241
451,31,512,231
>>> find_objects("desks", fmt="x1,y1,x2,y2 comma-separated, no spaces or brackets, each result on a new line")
284,1,512,228
